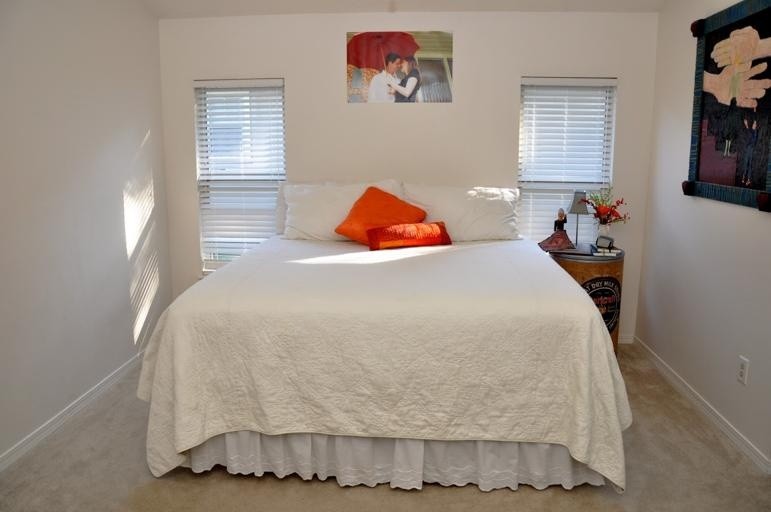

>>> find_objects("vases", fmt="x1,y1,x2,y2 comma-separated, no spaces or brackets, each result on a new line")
597,223,610,247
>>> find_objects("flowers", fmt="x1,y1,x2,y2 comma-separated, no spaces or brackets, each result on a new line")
577,190,630,225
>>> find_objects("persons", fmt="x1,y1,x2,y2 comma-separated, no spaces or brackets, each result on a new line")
366,52,401,103
389,55,423,102
538,208,575,253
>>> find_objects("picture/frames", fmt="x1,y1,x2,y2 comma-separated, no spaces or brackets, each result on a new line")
682,0,769,212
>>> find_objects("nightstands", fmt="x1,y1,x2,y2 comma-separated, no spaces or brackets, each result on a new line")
551,244,626,352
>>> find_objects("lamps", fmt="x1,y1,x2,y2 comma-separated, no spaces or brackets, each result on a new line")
568,190,589,245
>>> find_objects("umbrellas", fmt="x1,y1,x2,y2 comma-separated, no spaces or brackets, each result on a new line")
347,32,420,70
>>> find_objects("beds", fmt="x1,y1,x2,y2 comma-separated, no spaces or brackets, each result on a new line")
137,228,634,495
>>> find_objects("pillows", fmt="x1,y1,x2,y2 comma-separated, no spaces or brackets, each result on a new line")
280,181,401,242
336,186,426,247
404,182,521,241
370,221,451,250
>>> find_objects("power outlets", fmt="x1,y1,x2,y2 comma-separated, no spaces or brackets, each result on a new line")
736,355,749,384
